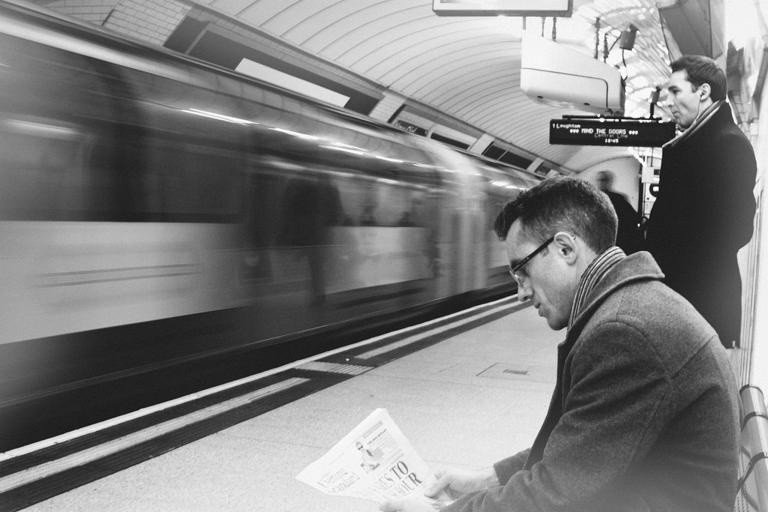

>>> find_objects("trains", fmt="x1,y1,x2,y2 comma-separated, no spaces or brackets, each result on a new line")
0,0,557,415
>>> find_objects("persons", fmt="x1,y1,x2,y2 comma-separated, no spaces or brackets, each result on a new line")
597,171,638,256
380,175,743,512
645,55,757,349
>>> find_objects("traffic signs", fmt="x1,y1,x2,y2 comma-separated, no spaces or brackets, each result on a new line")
548,116,676,146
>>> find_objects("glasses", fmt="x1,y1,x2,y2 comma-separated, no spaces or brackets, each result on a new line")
509,237,554,285
